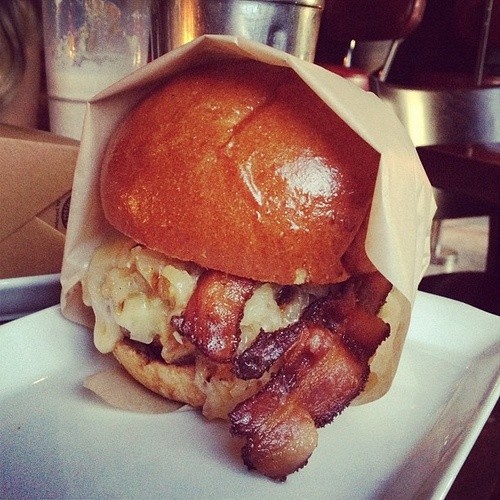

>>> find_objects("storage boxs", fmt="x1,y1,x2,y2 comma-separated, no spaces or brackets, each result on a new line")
0,124,82,280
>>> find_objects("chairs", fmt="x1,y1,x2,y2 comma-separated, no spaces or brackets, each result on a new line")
367,71,500,316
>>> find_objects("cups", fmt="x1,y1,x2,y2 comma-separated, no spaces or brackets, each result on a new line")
44,0,150,142
146,1,324,69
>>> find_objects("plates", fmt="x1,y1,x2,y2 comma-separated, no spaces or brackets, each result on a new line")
0,285,500,500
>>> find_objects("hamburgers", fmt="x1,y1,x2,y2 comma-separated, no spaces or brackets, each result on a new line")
78,61,391,481
